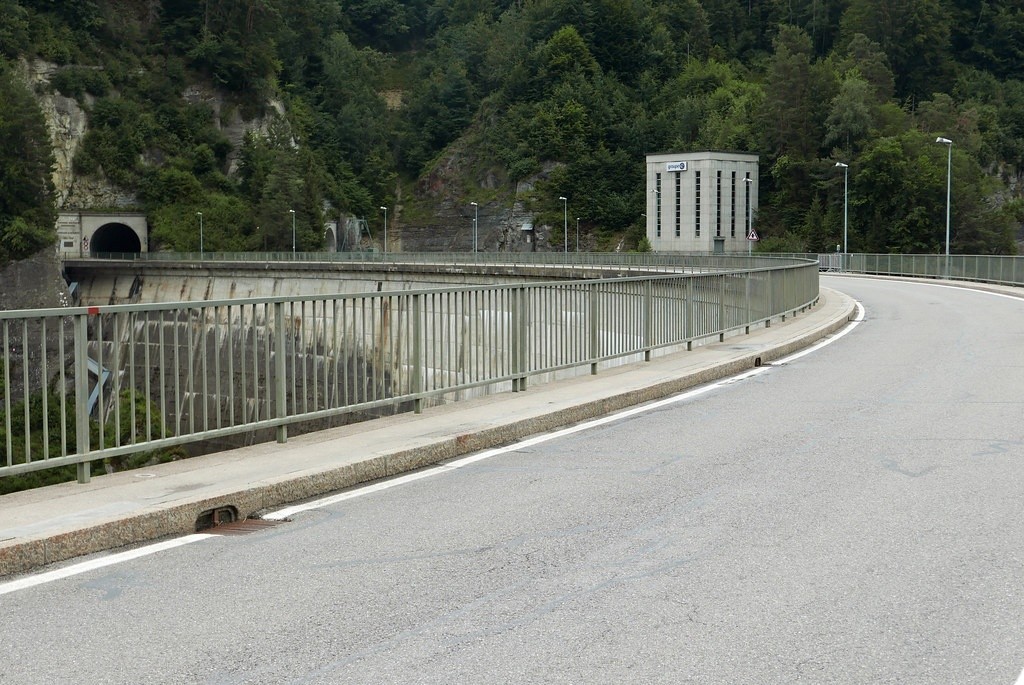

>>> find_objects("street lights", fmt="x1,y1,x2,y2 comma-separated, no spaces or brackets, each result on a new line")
196,211,203,261
380,206,388,263
742,177,752,269
834,162,848,271
576,217,580,263
935,136,953,280
559,197,568,264
290,209,296,260
472,218,476,258
470,202,478,263
